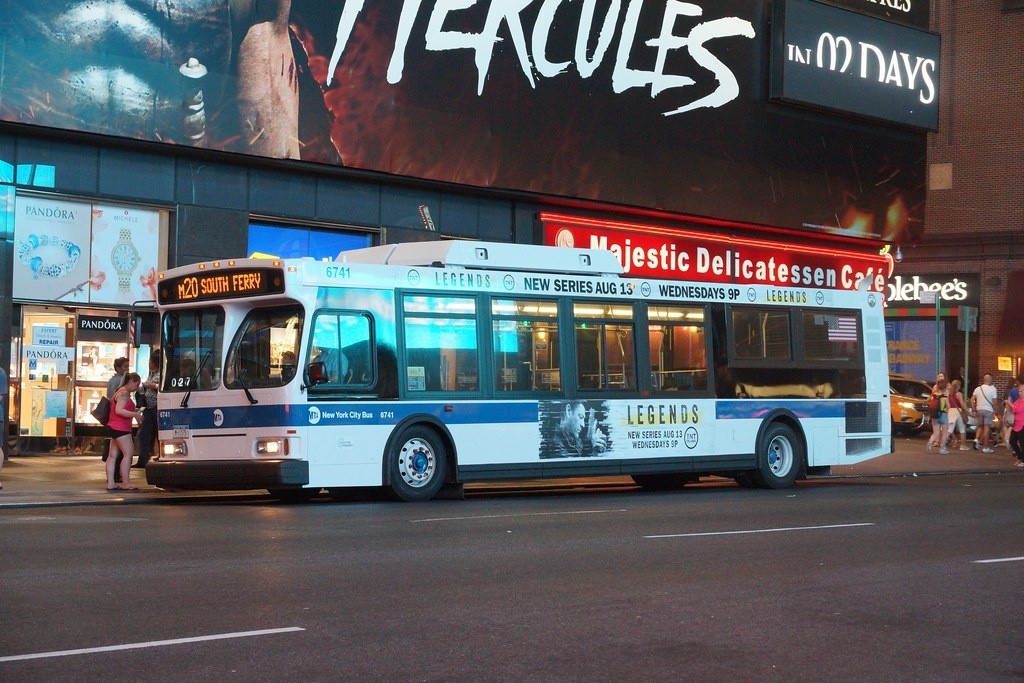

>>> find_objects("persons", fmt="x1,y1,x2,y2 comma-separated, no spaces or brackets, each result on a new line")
105,371,145,492
177,343,301,389
131,351,161,470
553,398,607,456
104,358,130,483
926,365,1024,467
0,365,8,490
312,329,349,383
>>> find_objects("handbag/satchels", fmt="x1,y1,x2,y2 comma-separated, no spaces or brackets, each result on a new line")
91,396,112,426
962,413,970,423
1003,404,1015,426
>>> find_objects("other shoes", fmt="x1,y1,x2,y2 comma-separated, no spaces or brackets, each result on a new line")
994,439,999,447
960,445,970,451
927,442,932,454
1014,459,1024,468
131,464,145,468
939,449,949,454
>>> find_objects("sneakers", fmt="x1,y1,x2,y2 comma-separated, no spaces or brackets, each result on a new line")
973,439,979,450
983,447,994,453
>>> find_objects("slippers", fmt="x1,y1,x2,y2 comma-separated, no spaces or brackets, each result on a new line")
106,486,122,490
122,486,139,490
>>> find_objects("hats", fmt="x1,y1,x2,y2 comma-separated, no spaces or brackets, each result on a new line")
183,351,195,361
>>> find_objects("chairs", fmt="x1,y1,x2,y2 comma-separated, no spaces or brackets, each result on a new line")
454,368,707,390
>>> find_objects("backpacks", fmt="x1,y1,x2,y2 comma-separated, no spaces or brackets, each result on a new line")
929,394,948,418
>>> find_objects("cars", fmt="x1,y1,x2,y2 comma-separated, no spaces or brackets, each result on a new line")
888,374,933,436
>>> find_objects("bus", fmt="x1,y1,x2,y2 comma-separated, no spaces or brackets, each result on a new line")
142,240,892,502
